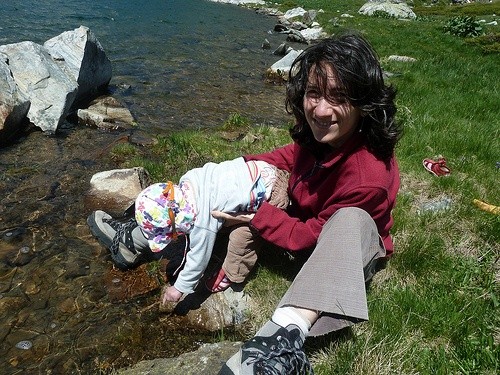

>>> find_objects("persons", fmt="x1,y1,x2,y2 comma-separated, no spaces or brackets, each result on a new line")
87,31,407,375
135,155,290,305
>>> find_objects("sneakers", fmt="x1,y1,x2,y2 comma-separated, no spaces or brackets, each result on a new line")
86,209,143,270
421,158,450,178
205,267,235,293
216,318,314,375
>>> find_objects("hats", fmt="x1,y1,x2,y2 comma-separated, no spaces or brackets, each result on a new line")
134,181,194,252
